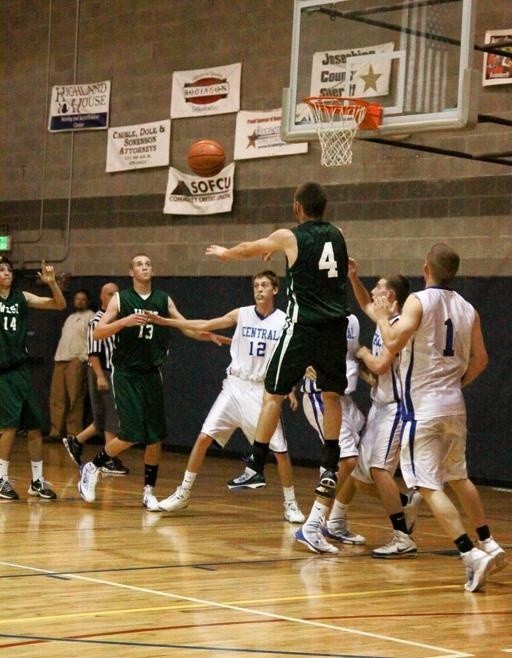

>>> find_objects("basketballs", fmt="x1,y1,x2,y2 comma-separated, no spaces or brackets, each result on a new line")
188,140,225,177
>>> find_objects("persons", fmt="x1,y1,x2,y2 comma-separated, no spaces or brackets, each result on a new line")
205,182,348,498
347,256,422,559
145,269,306,524
78,253,233,511
43,292,96,443
62,282,128,475
0,256,67,500
294,313,377,554
374,242,508,592
